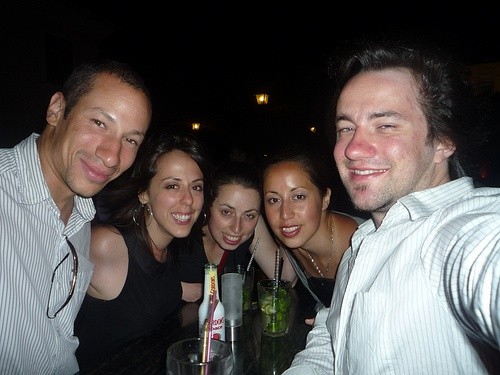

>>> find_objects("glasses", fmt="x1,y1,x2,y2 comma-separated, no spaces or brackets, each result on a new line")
46,233,78,319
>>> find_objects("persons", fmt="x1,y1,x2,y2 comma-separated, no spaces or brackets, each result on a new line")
180,173,261,302
0,62,152,375
86,142,204,375
280,47,500,375
249,154,369,325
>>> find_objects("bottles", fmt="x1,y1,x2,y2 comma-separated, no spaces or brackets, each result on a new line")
198,263,226,360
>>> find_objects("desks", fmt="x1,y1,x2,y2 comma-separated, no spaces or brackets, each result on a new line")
74,277,335,375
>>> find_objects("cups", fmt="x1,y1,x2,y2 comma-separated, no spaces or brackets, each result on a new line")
167,338,234,375
224,264,255,318
257,280,292,338
221,273,243,327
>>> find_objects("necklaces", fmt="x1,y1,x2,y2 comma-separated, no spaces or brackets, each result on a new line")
306,225,333,288
